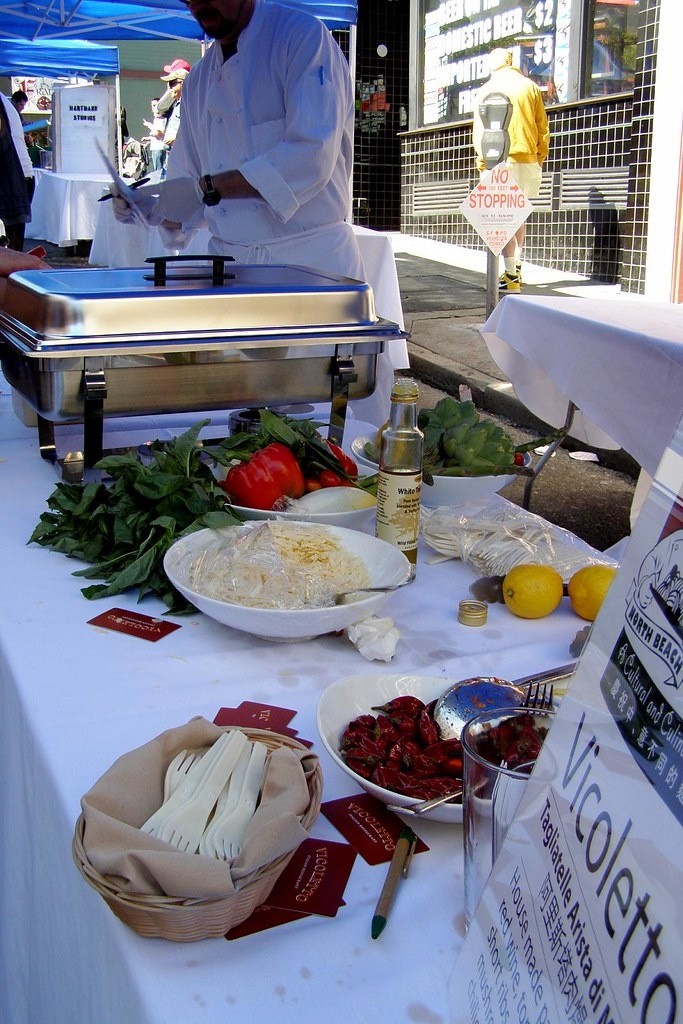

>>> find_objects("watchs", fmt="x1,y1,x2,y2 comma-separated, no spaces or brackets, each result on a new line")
202,175,221,208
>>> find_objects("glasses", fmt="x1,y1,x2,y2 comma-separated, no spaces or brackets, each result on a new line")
169,80,182,87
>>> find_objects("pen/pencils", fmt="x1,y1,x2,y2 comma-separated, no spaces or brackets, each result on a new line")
371,826,418,940
98,178,151,202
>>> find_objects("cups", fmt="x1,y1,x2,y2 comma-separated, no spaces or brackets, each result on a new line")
460,706,556,937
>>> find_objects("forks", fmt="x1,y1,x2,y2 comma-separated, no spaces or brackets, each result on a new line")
418,505,620,577
138,730,268,860
524,680,553,718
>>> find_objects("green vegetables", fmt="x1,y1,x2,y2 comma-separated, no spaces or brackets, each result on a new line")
24,408,378,615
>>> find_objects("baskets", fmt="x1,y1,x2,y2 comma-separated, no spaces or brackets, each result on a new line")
72,729,322,943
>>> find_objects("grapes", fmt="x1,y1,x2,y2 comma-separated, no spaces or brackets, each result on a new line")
470,574,506,604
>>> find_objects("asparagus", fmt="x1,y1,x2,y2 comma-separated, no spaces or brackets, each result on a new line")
354,443,447,495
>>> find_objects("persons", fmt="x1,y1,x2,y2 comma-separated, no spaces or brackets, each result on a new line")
110,0,363,281
114,59,191,186
0,89,40,252
0,245,53,279
473,48,550,293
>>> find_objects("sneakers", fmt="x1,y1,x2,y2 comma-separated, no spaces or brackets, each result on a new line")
483,273,521,293
515,264,522,285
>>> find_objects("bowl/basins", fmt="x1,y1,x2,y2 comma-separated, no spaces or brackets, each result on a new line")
316,673,462,824
225,503,376,531
350,431,532,510
163,520,412,644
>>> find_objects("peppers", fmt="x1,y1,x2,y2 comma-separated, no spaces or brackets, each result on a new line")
220,441,306,511
339,695,555,803
305,438,358,491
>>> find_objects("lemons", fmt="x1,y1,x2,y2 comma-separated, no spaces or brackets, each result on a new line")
568,565,614,621
503,564,564,618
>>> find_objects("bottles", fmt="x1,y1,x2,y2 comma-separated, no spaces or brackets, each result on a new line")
374,378,424,566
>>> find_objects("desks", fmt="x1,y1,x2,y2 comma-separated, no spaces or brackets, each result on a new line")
480,295,683,510
0,376,613,1024
87,188,411,371
23,168,135,254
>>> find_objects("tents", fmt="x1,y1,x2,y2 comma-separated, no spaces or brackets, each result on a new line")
0,0,359,177
530,42,629,95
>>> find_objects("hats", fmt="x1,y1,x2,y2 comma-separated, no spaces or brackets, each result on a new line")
488,48,512,71
159,68,189,81
163,58,190,73
